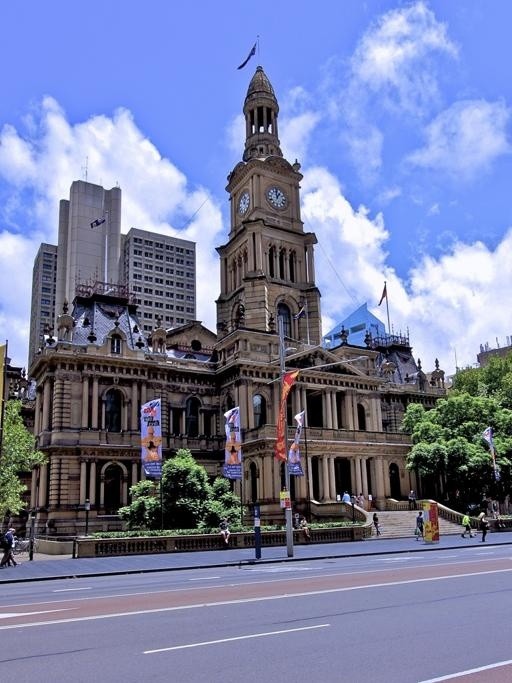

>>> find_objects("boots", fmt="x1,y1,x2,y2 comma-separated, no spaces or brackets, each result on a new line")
462,534,474,538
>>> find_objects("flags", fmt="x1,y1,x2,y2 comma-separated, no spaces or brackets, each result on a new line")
377,285,386,307
237,43,257,70
91,216,106,228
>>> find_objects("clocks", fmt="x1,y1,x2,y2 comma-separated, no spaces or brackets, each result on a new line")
237,188,250,218
265,183,289,210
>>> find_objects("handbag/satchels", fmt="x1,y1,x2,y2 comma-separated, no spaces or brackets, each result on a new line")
479,521,489,531
415,528,421,535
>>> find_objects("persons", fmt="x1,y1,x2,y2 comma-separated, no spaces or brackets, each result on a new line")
336,491,373,512
0,528,18,569
300,516,311,537
219,518,231,544
408,490,419,510
226,432,241,464
373,513,381,535
141,426,161,461
415,512,423,541
461,502,499,541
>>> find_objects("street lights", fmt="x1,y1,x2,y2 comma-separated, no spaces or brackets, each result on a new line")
85,498,91,536
30,511,35,560
277,315,370,558
351,495,355,523
